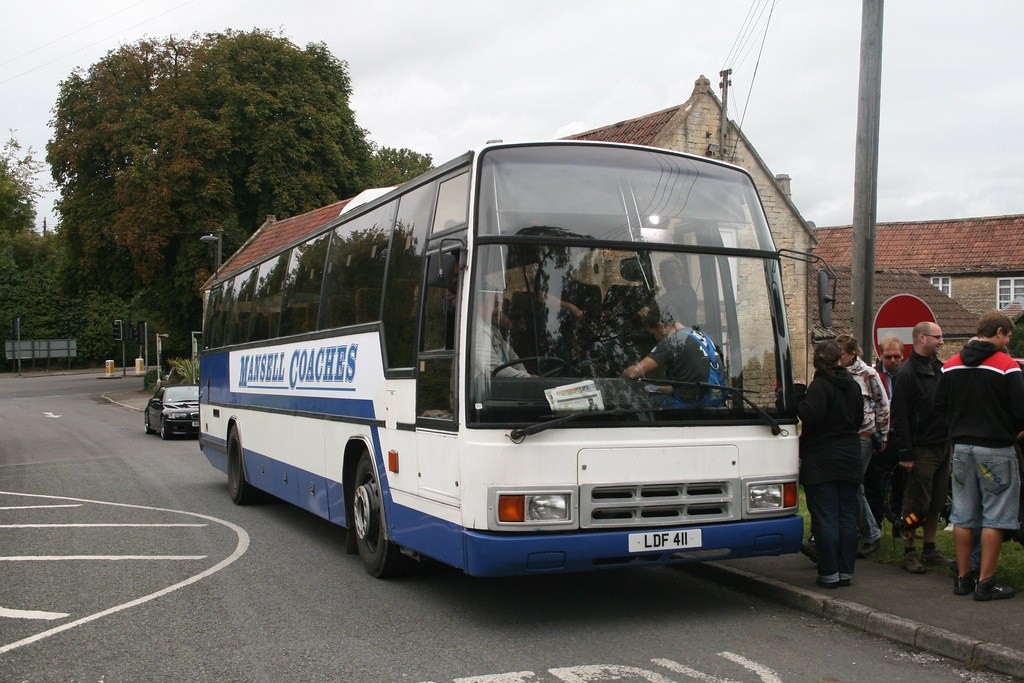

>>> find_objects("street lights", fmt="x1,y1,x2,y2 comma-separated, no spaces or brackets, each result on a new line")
156,333,170,388
201,235,221,286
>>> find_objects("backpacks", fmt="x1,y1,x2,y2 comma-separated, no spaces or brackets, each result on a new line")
686,333,728,407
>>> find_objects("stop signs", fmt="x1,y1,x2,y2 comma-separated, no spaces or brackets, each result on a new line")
871,293,938,371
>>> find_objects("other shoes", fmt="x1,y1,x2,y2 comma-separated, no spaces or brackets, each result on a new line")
801,540,818,562
816,576,851,588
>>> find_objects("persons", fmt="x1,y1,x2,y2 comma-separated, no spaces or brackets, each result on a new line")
794,340,864,589
800,321,951,573
939,311,1024,602
472,248,728,406
410,255,459,316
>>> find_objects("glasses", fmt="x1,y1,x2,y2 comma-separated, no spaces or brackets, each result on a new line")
924,334,941,340
881,353,902,359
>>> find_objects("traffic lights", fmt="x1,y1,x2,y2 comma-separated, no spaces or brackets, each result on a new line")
113,320,122,339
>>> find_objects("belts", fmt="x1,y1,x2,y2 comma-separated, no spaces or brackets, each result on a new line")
858,431,872,439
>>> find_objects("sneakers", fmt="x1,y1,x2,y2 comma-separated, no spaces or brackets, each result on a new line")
899,551,927,573
973,574,1015,600
953,569,977,595
859,538,881,557
920,549,945,563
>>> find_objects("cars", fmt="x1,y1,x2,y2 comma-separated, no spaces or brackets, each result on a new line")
144,383,200,440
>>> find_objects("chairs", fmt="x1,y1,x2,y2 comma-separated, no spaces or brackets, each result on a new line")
208,280,648,369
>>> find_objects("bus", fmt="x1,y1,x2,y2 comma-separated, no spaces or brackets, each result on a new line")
198,139,839,583
